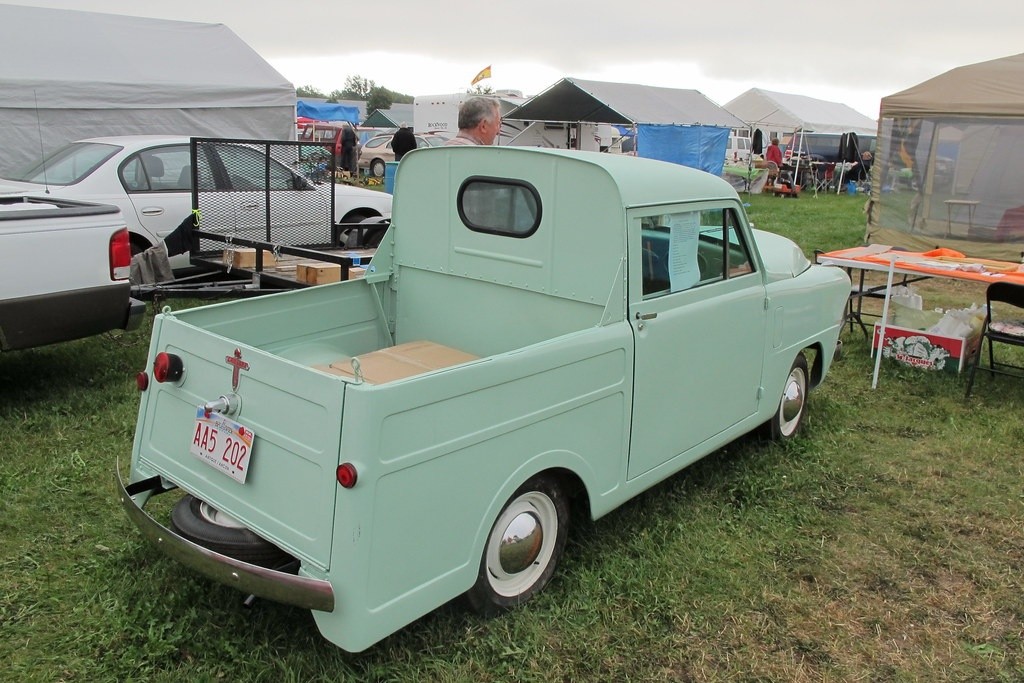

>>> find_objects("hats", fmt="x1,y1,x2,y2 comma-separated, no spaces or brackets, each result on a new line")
342,122,349,126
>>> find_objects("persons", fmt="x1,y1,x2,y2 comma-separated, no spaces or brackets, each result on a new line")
766,138,783,167
852,151,872,181
341,122,356,170
440,97,502,226
391,121,417,161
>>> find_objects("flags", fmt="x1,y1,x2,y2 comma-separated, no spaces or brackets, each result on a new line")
471,66,491,85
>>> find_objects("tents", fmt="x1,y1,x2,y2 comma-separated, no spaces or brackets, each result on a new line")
722,88,879,194
297,100,360,182
498,77,754,196
0,4,299,173
864,52,1024,264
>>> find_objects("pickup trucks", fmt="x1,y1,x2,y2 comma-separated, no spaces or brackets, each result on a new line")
114,144,853,654
0,195,148,366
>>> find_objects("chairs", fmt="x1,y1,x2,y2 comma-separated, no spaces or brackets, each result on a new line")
963,281,1024,402
848,247,907,332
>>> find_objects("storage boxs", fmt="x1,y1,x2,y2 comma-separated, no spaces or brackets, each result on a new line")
221,248,276,269
871,312,982,374
295,263,343,284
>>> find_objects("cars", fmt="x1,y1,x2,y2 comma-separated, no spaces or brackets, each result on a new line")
297,116,449,179
0,135,392,359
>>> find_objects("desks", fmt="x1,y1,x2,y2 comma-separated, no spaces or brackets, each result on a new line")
815,245,1024,391
791,162,858,198
942,199,984,237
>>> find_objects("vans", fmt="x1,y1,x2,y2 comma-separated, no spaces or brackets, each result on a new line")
785,132,877,173
